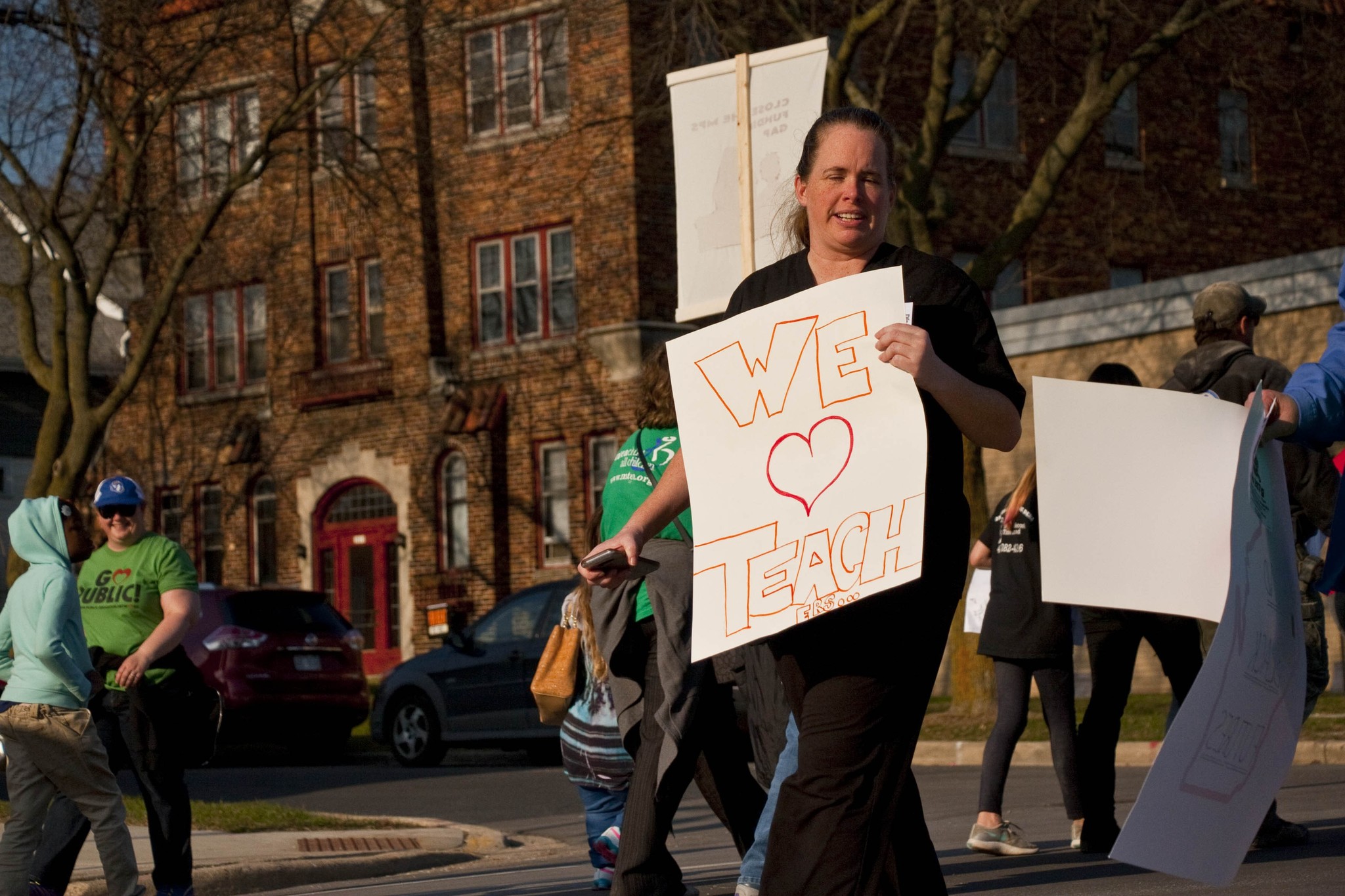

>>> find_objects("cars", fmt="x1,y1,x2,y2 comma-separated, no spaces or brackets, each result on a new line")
373,577,579,768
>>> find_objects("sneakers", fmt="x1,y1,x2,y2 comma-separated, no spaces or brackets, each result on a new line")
1071,821,1085,846
1079,815,1123,858
595,824,622,862
967,821,1038,855
591,866,617,890
1244,815,1313,851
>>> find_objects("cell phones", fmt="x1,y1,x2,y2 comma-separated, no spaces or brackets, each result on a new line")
581,547,661,582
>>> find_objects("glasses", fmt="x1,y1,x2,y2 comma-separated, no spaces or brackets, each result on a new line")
99,504,136,519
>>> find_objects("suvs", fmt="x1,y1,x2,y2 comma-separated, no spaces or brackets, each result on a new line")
191,583,371,766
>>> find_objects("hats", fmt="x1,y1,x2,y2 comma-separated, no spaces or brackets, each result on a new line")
91,474,147,510
1192,280,1270,328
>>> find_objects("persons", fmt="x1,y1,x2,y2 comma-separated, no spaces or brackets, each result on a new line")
77,477,196,896
559,507,635,891
579,106,1028,895
1079,362,1206,865
966,461,1087,857
1243,260,1343,632
1150,279,1344,861
0,496,138,896
599,339,770,896
